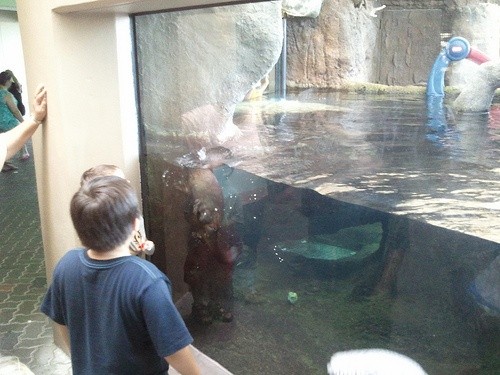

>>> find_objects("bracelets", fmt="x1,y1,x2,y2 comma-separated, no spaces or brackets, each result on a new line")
30,114,43,125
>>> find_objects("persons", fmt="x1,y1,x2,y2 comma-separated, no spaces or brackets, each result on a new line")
38,164,215,375
0,84,46,173
0,70,32,173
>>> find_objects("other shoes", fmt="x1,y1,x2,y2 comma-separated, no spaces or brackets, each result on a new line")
2,162,18,172
21,153,29,160
